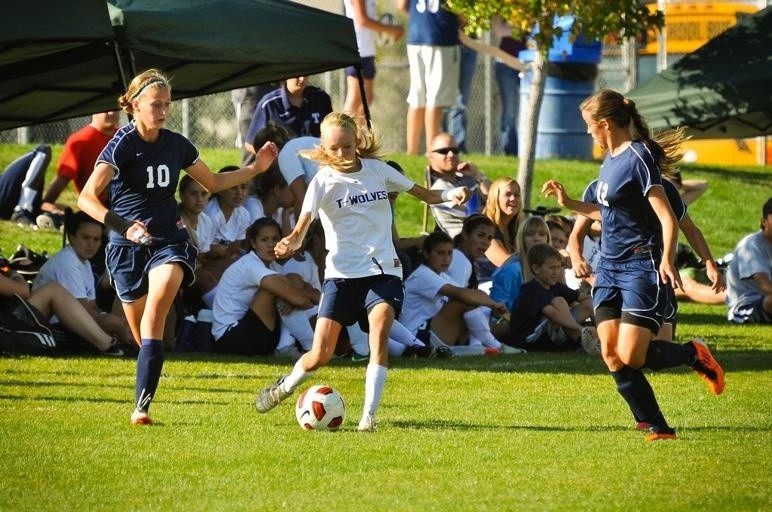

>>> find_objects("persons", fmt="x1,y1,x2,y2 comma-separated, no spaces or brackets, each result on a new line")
0,123,727,363
341,0,405,139
725,197,772,325
228,83,272,164
440,11,526,157
76,68,278,426
542,89,724,441
256,112,473,433
42,111,120,206
395,0,462,160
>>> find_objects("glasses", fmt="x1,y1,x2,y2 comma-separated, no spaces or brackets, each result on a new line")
431,146,460,156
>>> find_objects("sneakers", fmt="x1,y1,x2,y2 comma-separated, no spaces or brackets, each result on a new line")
357,414,377,431
580,325,602,357
688,336,726,396
634,423,677,443
130,409,152,425
497,345,528,356
273,345,454,366
9,205,64,234
96,335,139,358
254,373,295,414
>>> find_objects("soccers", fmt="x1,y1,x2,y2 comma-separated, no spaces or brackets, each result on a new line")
296,384,345,430
